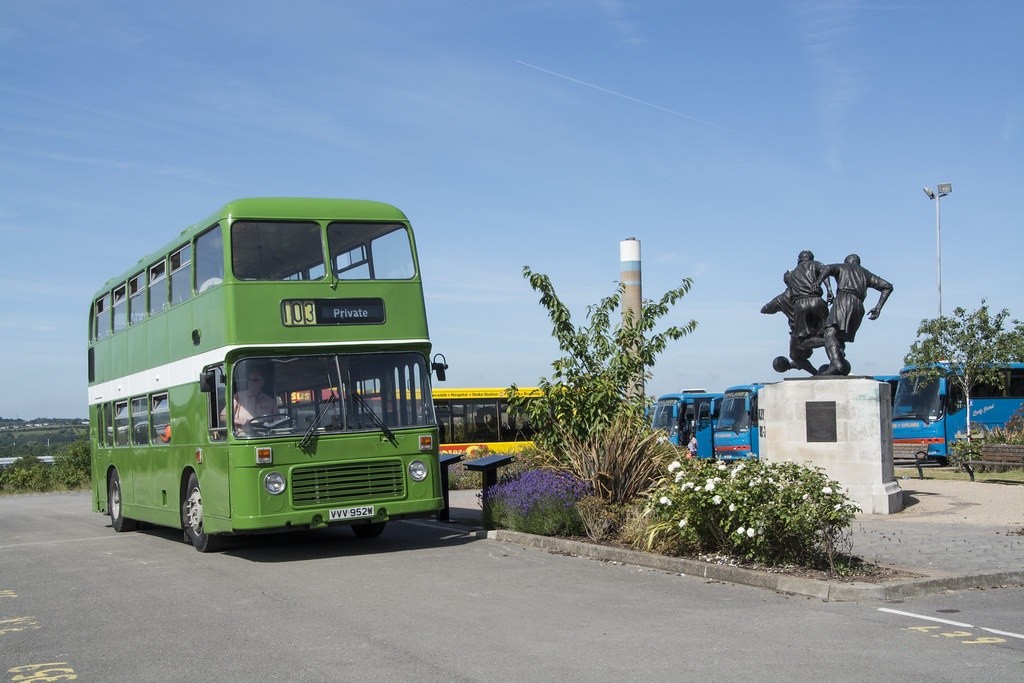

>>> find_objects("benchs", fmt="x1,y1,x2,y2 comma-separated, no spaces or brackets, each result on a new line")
893,443,930,480
961,445,1024,482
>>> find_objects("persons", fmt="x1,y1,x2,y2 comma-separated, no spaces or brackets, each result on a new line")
761,250,894,374
687,433,697,456
219,372,276,435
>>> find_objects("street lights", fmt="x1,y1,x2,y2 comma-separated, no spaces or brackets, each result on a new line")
923,184,952,345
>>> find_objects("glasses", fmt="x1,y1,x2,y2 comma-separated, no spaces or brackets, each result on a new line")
248,377,258,383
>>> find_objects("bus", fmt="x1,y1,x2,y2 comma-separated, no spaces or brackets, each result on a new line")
892,363,1024,465
649,375,898,467
86,197,449,552
281,387,569,459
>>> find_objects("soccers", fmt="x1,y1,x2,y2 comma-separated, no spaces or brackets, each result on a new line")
773,356,852,375
